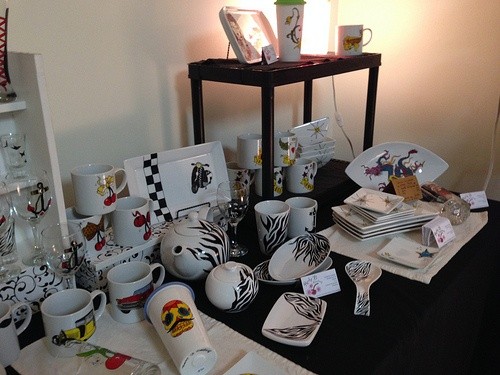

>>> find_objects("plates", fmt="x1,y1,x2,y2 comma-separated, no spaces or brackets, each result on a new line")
121,140,231,227
332,188,437,242
262,292,327,346
268,233,332,281
253,256,333,284
345,141,449,192
220,8,279,64
376,237,441,271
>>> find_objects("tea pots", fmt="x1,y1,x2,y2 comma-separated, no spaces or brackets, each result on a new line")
160,206,230,279
206,261,259,312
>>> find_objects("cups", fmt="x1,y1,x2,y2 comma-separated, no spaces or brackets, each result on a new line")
338,25,372,56
274,131,299,167
227,161,283,205
286,158,318,193
144,281,217,375
255,200,291,258
237,134,262,169
285,197,317,241
63,163,156,259
274,0,306,62
0,133,29,180
0,261,165,367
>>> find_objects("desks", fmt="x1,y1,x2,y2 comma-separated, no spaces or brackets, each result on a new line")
187,52,383,200
0,160,499,374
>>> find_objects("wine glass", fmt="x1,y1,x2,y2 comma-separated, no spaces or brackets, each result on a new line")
0,169,87,290
216,182,250,257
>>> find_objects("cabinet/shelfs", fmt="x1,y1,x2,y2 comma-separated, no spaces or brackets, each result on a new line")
0,52,74,323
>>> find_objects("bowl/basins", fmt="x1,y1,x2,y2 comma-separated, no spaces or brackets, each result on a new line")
288,117,334,168
